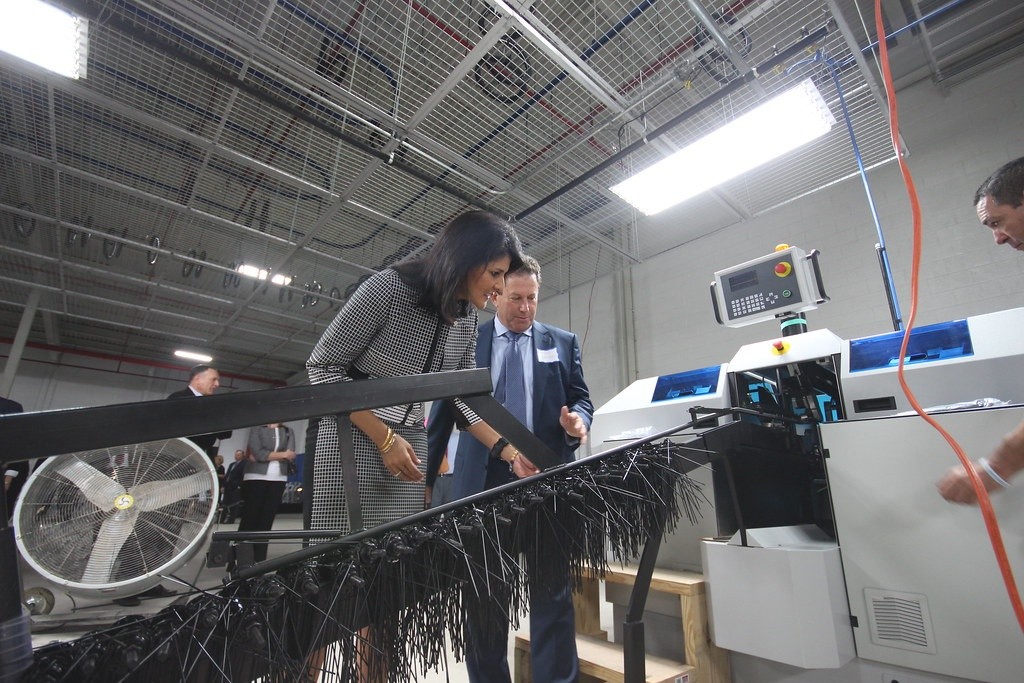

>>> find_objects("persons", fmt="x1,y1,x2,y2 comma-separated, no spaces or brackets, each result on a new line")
111,365,296,606
426,255,594,683
306,210,539,683
0,397,29,525
936,156,1024,506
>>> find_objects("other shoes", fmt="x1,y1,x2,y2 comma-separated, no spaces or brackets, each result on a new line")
112,596,140,606
138,585,178,596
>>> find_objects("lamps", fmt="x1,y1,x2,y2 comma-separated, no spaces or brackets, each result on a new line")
0,1,90,80
607,74,838,216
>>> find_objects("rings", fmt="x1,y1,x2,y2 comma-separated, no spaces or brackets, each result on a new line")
396,471,401,477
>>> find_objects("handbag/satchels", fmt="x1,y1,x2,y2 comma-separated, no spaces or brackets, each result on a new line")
288,459,299,475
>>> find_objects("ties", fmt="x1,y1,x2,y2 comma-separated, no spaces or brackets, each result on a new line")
493,331,528,429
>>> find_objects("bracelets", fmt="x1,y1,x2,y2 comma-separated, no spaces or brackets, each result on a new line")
509,450,518,472
978,458,1010,488
379,426,396,454
491,437,509,459
